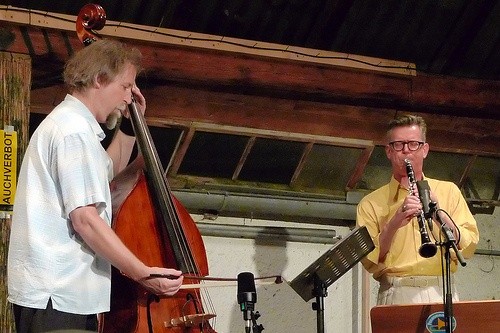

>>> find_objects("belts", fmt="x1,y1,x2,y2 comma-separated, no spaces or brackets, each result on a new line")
376,275,453,288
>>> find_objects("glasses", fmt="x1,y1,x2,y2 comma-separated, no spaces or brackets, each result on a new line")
388,140,426,153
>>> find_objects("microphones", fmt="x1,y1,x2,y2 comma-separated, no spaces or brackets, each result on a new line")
416,181,433,231
237,272,256,333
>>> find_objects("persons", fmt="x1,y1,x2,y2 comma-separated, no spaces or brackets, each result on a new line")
7,39,184,333
356,113,479,306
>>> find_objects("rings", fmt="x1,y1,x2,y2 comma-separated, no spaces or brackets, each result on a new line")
402,205,406,211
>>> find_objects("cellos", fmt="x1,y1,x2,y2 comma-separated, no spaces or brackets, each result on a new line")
75,4,217,333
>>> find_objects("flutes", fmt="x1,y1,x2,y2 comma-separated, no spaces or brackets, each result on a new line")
405,159,438,258
